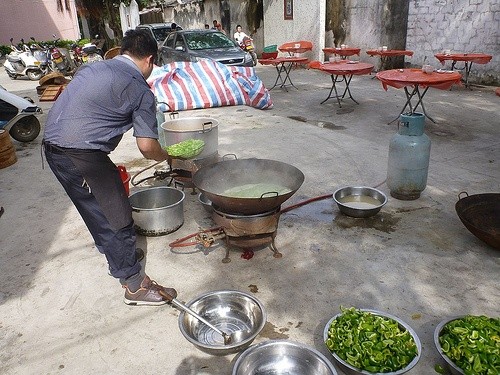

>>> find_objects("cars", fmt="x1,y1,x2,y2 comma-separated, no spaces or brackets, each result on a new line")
136,24,183,49
156,30,255,66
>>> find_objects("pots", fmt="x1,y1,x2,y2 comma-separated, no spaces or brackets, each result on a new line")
159,113,220,157
455,190,500,250
192,153,305,216
128,181,186,237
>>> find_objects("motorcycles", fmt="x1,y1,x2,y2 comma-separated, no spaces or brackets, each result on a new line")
235,35,259,63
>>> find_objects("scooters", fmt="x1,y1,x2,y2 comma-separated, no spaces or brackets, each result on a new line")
4,38,103,81
0,85,43,142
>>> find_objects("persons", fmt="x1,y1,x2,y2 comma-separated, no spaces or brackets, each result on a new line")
205,20,226,35
233,24,253,45
41,29,178,306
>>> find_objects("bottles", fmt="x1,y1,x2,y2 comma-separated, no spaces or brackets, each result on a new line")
340,44,346,49
442,49,451,56
422,65,433,75
328,57,340,64
294,43,300,48
379,46,387,53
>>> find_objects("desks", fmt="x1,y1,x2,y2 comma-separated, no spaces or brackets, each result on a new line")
322,48,360,60
435,53,492,91
312,61,373,108
278,41,313,60
258,58,308,92
367,50,412,78
377,69,461,126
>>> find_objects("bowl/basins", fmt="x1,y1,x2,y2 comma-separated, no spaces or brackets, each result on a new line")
179,290,267,356
262,44,279,60
333,185,389,217
323,308,422,375
231,339,339,375
434,313,467,375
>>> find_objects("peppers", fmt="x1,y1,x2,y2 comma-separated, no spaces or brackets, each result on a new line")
164,138,205,159
434,315,500,375
325,305,419,374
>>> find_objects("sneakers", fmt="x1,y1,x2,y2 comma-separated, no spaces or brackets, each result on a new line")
123,275,177,307
110,248,144,278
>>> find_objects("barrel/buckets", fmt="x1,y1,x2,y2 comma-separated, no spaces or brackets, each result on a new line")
116,165,130,197
